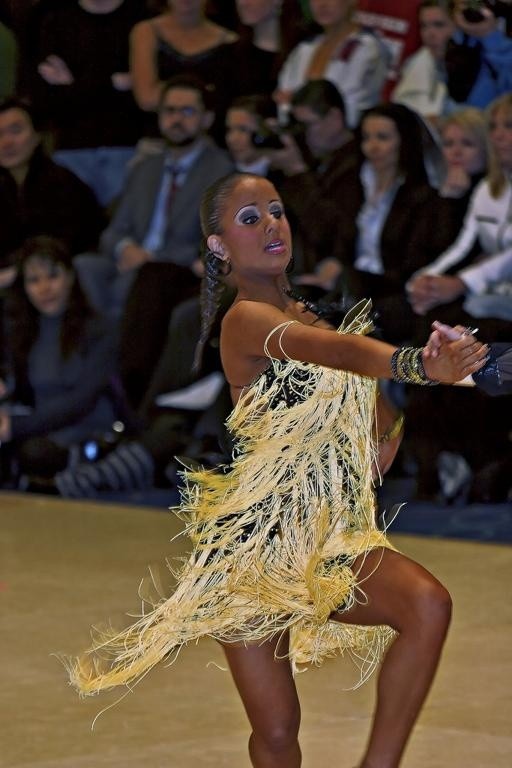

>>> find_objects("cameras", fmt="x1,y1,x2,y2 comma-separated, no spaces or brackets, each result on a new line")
250,110,310,150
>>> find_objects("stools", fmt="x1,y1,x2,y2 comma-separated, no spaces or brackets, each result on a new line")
410,420,512,503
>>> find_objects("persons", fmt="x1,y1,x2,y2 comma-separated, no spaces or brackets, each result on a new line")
0,0,509,549
191,170,490,768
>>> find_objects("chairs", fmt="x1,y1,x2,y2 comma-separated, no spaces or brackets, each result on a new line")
57,148,132,212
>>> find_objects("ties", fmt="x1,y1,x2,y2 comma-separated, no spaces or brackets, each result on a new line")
167,166,181,212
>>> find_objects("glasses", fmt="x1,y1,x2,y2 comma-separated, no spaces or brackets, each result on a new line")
158,106,202,118
